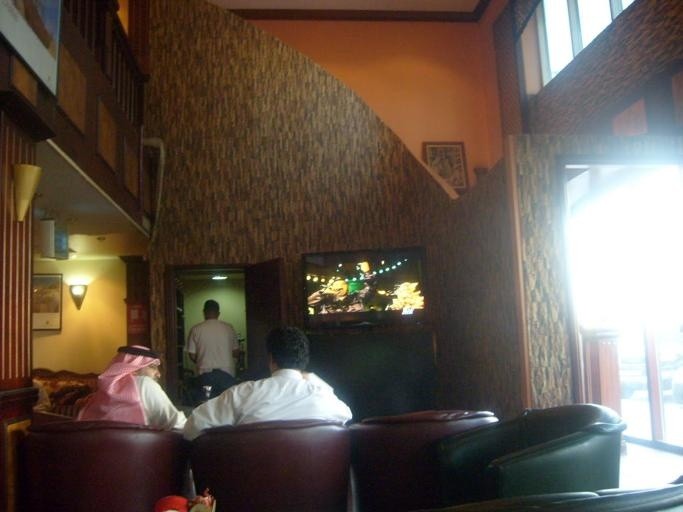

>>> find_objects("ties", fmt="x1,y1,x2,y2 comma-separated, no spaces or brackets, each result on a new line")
297,246,431,338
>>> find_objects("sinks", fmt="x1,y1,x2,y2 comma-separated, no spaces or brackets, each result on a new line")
353,409,496,512
13,421,179,510
440,475,682,511
433,400,627,491
194,418,351,512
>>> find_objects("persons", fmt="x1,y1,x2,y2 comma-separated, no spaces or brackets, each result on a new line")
76,345,187,429
184,299,240,399
180,321,352,441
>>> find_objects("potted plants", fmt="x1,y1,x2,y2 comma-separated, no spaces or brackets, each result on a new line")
31,272,63,332
421,141,469,192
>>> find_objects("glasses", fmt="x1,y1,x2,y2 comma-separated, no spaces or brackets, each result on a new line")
12,164,41,222
71,285,88,311
